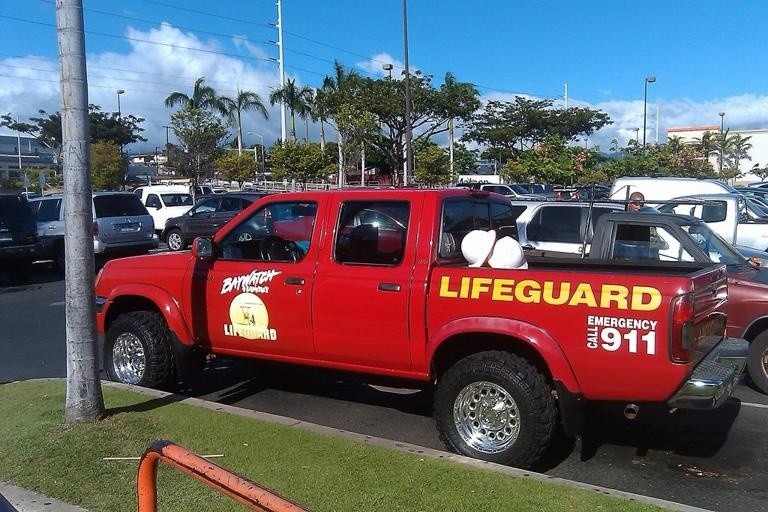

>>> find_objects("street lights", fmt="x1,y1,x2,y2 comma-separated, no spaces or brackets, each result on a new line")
117,90,124,153
719,112,725,171
644,76,655,147
248,131,264,155
383,64,399,186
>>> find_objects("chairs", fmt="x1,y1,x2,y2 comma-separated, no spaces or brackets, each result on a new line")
340,222,380,262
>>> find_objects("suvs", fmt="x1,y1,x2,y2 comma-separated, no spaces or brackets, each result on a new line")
28,190,159,274
132,184,214,235
455,175,768,397
0,191,44,282
159,190,300,251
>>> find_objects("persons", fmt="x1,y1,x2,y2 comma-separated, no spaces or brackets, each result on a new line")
627,192,644,213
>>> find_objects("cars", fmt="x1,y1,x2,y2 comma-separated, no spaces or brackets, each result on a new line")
272,203,410,255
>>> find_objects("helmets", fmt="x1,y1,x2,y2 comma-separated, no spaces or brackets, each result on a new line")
486,235,532,270
458,227,497,268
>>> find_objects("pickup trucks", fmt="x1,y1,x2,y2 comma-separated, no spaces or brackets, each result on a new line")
94,184,752,470
